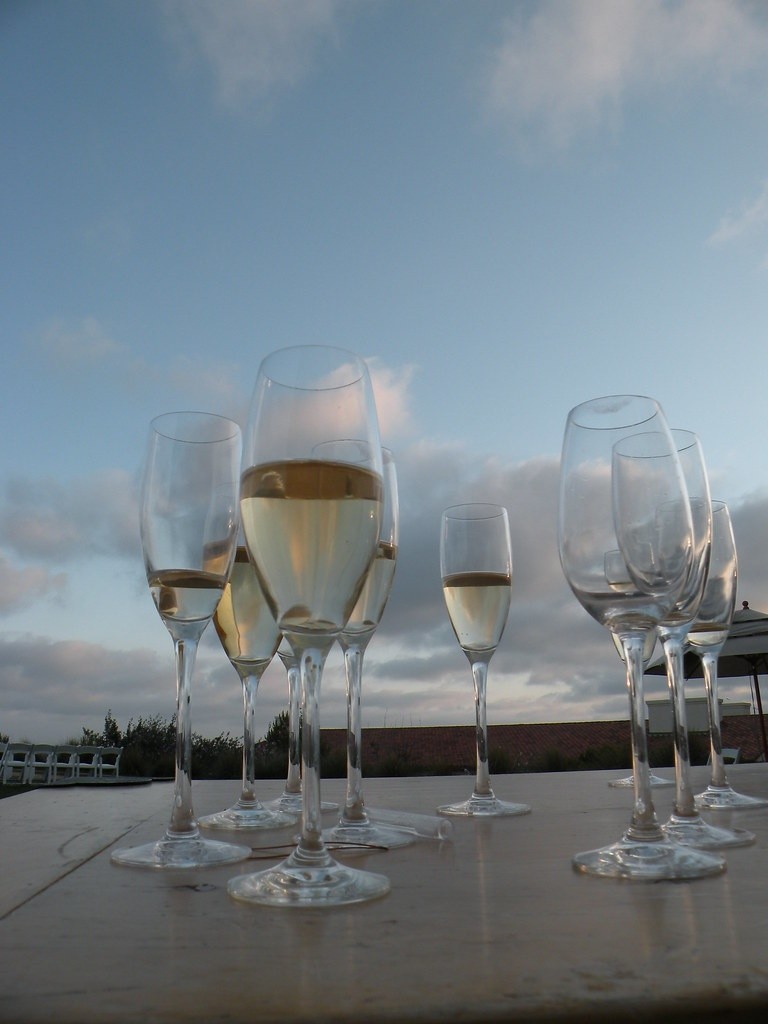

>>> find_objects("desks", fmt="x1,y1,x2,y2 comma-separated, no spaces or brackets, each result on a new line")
0,761,768,1024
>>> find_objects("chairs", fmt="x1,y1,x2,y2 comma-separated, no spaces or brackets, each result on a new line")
3,743,32,785
27,744,55,784
77,746,101,777
51,744,79,783
99,744,124,779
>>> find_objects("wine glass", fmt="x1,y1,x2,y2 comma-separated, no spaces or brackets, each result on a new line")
197,480,299,833
613,428,757,852
655,499,768,811
111,412,253,869
224,344,390,906
256,630,340,815
555,395,728,881
603,548,678,789
437,502,533,818
294,439,419,851
